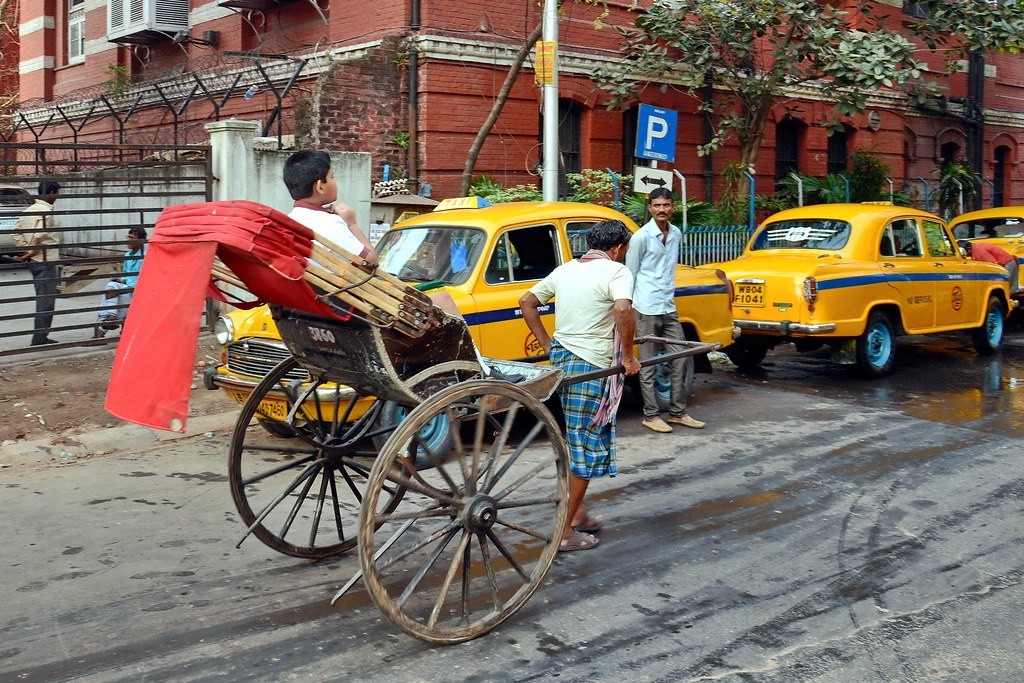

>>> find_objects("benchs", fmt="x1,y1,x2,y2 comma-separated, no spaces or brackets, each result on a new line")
268,293,489,390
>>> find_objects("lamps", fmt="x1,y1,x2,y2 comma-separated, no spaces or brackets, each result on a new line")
173,29,219,45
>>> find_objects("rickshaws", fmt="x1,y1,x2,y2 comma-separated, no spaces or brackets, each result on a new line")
144,201,724,645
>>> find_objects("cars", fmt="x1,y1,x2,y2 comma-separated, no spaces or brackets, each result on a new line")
696,199,1018,381
203,199,742,442
946,205,1024,296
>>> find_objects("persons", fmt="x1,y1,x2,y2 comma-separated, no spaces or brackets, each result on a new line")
283,150,525,385
626,187,707,433
11,180,61,345
956,240,1019,297
83,228,148,346
517,220,642,551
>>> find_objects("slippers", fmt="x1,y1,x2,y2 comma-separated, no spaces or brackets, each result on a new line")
556,514,605,552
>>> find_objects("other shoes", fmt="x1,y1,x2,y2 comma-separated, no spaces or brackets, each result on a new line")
101,316,121,330
82,337,109,348
667,413,705,429
30,337,58,346
642,416,674,434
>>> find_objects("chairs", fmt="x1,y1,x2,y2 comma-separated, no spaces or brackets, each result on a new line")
879,236,892,256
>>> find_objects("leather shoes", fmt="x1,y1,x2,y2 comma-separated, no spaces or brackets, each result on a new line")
477,364,526,386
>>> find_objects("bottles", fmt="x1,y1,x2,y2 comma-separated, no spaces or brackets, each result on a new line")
243,85,258,102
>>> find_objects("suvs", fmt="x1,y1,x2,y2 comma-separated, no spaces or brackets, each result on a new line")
0,184,37,256
